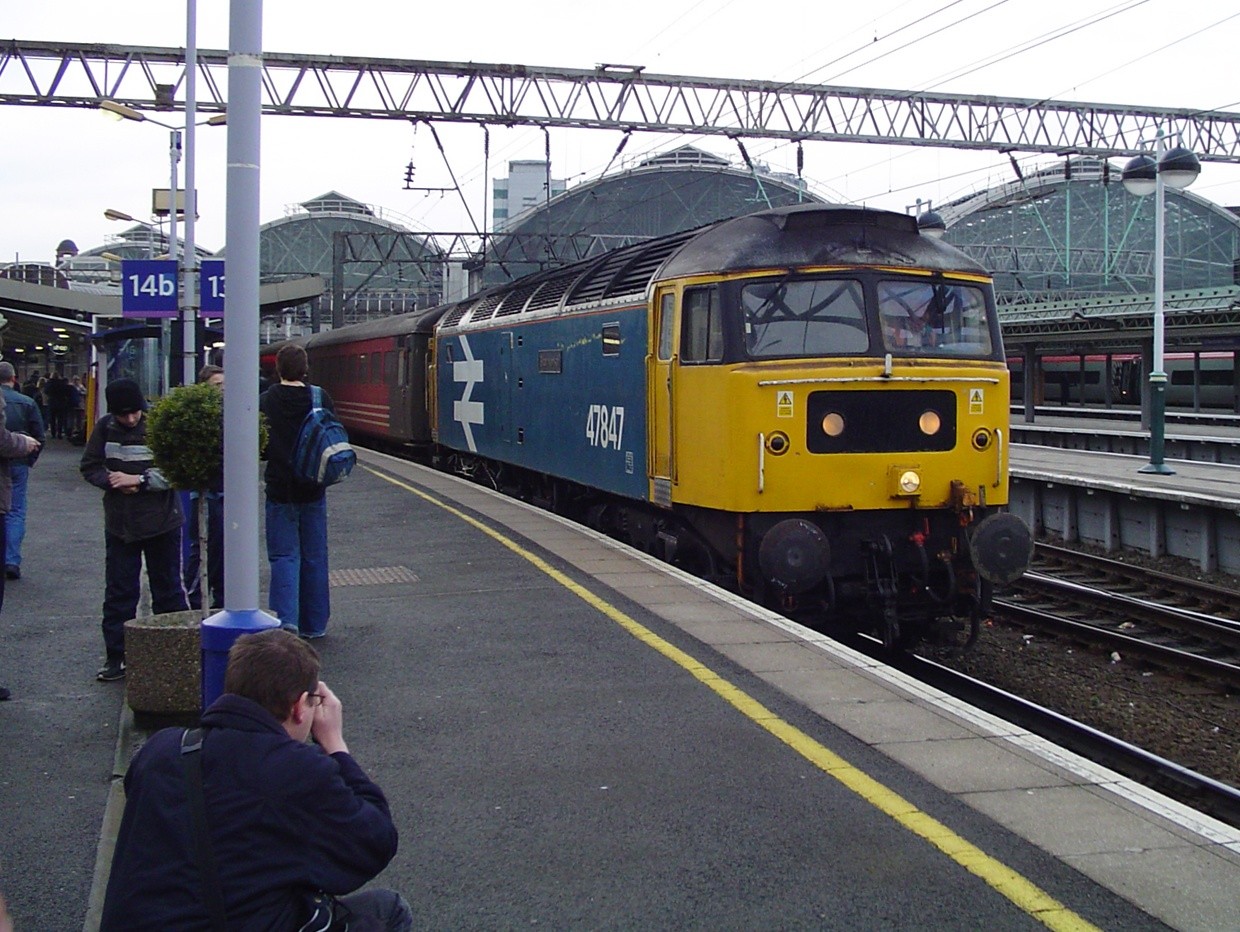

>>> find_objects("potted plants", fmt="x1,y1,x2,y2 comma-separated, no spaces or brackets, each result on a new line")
121,384,277,728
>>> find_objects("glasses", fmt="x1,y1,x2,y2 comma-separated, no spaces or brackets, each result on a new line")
308,694,325,706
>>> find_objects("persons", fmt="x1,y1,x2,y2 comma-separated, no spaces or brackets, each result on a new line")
14,370,87,439
81,378,188,680
182,365,224,610
99,628,413,932
891,301,936,350
0,362,46,578
0,338,42,703
260,343,338,640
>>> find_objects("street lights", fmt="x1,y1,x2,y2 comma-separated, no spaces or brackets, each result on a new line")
1119,130,1202,476
105,209,199,397
99,99,227,384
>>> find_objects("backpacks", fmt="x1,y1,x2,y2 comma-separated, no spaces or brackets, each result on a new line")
282,384,356,487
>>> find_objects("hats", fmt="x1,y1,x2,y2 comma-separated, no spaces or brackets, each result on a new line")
73,374,80,380
105,377,148,414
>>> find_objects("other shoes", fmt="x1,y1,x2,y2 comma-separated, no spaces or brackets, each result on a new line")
96,658,126,681
0,687,11,700
6,564,21,578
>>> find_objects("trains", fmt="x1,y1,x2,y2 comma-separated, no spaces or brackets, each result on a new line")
1005,352,1235,408
260,201,1035,658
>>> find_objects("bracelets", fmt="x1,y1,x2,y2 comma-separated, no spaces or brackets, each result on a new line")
138,474,144,487
